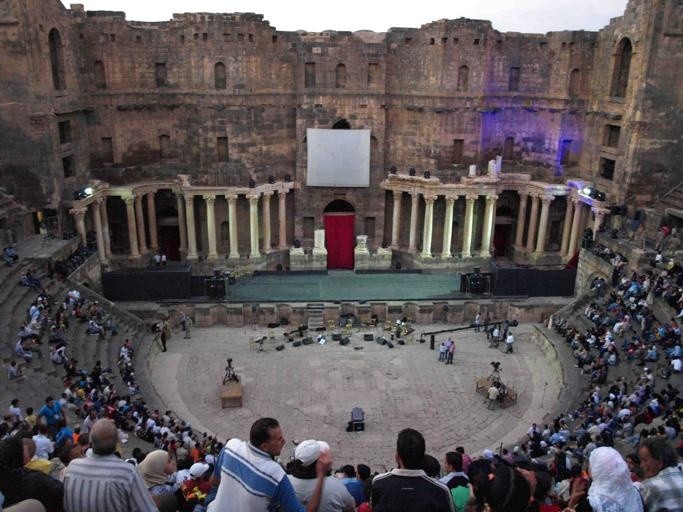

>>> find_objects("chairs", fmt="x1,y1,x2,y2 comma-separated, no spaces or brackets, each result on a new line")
351,407,365,431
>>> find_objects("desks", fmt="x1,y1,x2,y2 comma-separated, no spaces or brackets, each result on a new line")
475,378,517,409
221,376,243,409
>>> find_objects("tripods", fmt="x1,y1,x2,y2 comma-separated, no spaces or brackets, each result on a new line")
489,369,502,384
223,364,239,385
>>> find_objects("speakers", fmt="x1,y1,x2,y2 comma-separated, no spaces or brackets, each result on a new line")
206,280,216,298
461,273,491,297
216,280,225,298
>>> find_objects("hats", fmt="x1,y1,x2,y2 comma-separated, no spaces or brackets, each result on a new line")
295,438,330,467
190,462,210,479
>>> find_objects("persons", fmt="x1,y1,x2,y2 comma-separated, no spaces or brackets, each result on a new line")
438,336,455,365
475,311,513,353
1,244,120,383
151,310,192,352
402,316,408,333
543,223,682,372
1,367,683,511
153,251,167,266
